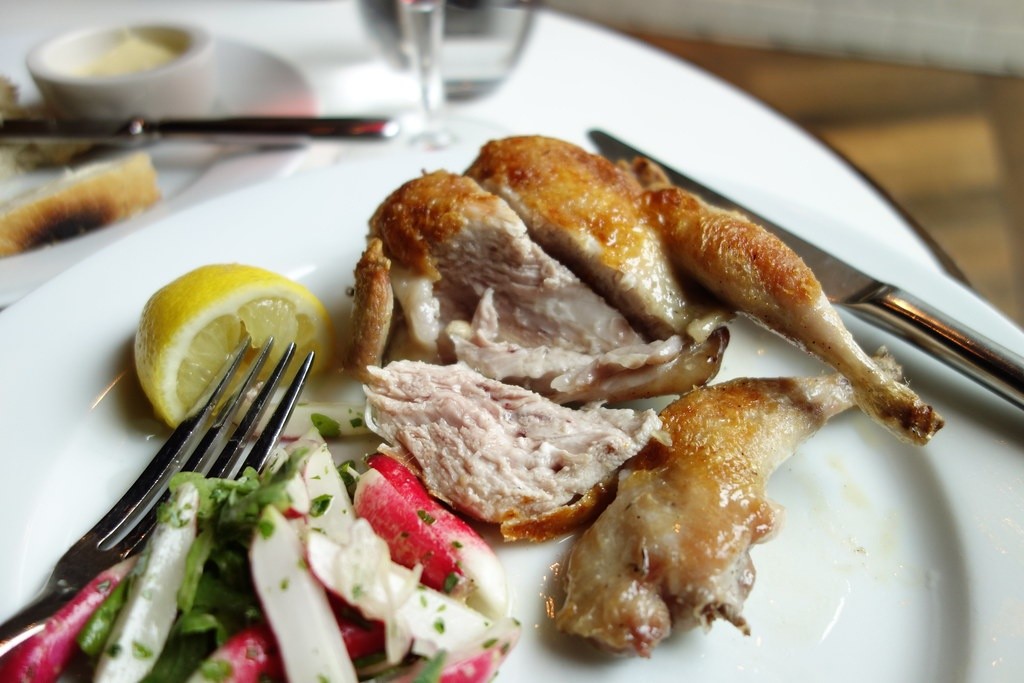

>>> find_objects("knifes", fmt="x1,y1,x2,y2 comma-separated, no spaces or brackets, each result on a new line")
0,117,400,145
587,128,1024,410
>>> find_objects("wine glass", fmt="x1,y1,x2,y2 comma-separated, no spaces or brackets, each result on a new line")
1,156,1023,682
355,0,543,152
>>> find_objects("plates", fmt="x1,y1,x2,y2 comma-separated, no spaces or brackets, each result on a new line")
0,33,311,149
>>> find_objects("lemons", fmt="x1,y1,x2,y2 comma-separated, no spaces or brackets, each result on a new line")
134,262,333,430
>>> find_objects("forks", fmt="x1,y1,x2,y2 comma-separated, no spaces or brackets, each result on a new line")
1,333,315,657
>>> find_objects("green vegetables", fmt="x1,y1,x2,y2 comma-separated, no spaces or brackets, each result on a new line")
71,412,522,683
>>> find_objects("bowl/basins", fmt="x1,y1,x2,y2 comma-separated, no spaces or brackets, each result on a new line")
29,16,213,117
